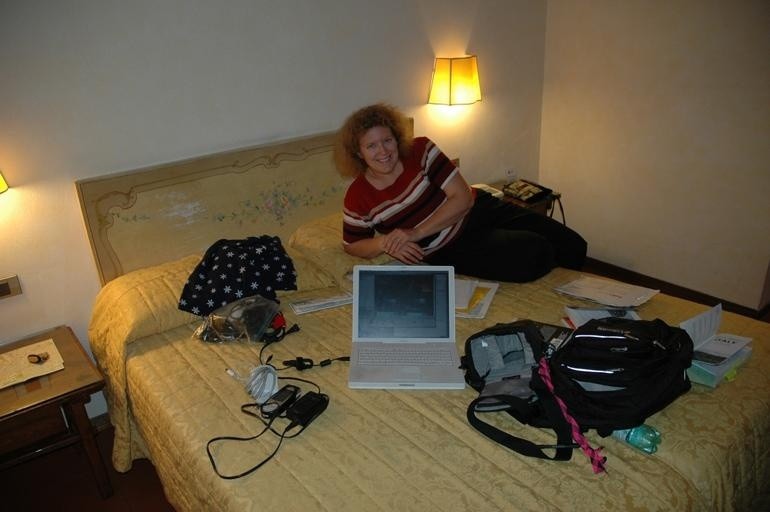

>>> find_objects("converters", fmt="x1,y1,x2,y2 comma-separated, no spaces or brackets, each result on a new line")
285,391,327,426
250,364,278,400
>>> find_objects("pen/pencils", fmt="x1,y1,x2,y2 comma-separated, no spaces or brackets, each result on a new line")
723,358,745,380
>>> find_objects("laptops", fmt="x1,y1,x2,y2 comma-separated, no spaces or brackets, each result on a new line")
348,264,466,390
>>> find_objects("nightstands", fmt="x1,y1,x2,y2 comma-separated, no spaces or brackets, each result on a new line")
0,325,114,503
488,175,561,226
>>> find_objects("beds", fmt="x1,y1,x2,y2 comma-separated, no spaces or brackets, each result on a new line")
74,117,769,510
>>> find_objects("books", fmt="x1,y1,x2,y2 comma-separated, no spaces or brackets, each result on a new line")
455,279,480,310
686,347,754,389
679,301,755,376
564,302,640,326
284,285,354,316
455,281,500,319
561,317,574,329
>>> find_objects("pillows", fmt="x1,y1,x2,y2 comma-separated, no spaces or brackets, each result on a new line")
110,213,397,343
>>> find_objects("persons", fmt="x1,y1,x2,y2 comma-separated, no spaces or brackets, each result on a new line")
338,104,588,284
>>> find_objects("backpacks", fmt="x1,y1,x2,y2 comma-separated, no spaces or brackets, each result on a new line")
460,318,579,462
548,316,695,437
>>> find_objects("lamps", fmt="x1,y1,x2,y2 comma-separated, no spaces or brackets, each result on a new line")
427,54,483,107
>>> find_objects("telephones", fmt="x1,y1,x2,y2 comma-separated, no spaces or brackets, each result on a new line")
471,184,504,198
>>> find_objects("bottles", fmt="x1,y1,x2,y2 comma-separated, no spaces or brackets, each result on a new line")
612,424,662,455
